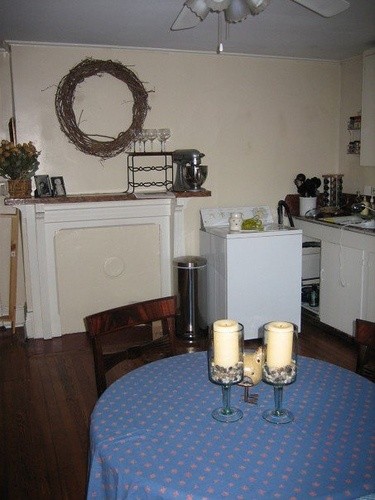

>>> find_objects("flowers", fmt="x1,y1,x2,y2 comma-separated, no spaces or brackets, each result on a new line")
0,140,41,178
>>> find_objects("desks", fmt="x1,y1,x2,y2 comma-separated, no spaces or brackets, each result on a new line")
87,350,375,499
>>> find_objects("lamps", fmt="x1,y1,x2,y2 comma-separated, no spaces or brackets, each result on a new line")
206,0,233,52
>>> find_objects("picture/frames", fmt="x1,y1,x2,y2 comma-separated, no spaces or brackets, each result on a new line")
33,175,53,197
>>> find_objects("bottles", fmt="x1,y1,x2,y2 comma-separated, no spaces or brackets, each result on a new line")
348,140,360,154
228,211,243,231
361,196,369,216
348,115,361,130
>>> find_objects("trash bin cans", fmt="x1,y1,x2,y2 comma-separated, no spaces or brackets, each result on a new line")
173,255,207,339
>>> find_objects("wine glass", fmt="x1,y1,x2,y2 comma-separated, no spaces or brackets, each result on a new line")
262,321,298,425
130,128,171,153
206,322,245,423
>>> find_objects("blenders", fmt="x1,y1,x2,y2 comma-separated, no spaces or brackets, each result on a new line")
171,148,205,192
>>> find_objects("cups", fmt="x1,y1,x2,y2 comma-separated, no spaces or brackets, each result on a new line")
299,197,317,216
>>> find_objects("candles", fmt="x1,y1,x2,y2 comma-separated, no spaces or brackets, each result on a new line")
212,320,238,370
266,320,293,372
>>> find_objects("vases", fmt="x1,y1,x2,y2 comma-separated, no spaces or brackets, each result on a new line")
8,178,31,198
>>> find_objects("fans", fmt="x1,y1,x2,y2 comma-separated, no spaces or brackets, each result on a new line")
171,0,353,31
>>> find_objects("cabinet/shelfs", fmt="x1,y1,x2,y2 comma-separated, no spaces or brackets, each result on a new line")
290,219,375,343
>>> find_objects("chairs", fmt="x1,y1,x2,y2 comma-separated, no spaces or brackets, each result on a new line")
83,295,182,398
353,318,375,385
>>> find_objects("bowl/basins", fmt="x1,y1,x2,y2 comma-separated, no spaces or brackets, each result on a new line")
185,165,208,191
